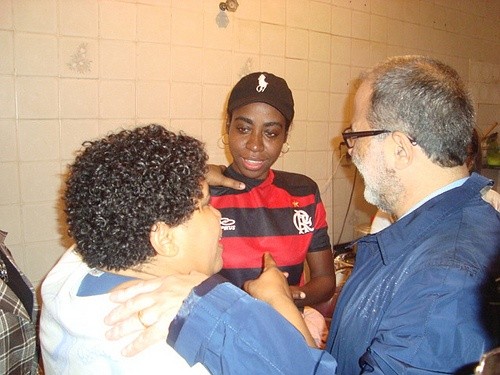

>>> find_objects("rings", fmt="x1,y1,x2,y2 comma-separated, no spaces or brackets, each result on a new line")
137,311,148,329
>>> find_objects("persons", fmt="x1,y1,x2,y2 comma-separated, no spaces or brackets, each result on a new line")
103,53,500,375
207,73,336,315
368,128,500,234
39,124,336,375
0,229,44,375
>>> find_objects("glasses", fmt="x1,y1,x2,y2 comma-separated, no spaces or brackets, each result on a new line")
343,122,417,148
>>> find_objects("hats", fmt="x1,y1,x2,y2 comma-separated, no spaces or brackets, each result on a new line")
227,72,294,124
